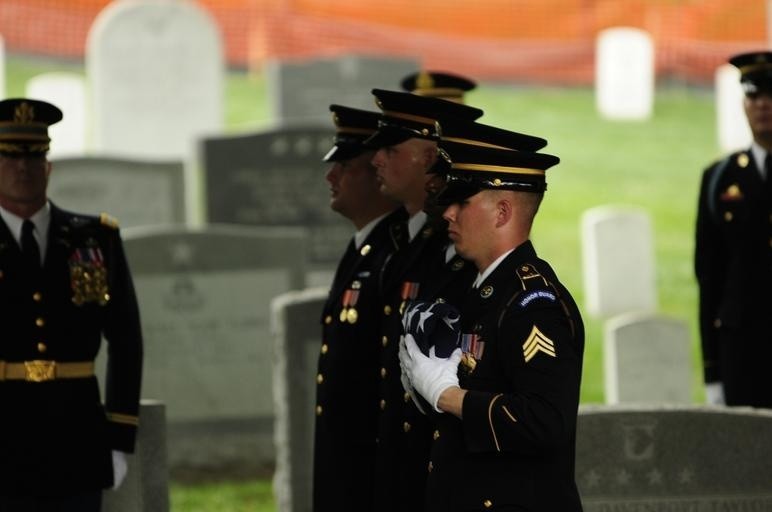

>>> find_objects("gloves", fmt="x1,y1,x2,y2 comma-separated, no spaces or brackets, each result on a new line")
706,382,727,406
102,449,128,492
397,333,464,415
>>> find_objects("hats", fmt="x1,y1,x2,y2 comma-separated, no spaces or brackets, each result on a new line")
361,88,483,152
730,51,772,93
426,118,547,177
405,71,477,101
322,105,383,162
433,142,560,206
0,98,62,155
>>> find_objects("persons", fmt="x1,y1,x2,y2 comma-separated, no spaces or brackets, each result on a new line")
308,66,588,511
1,96,146,510
690,48,770,413
